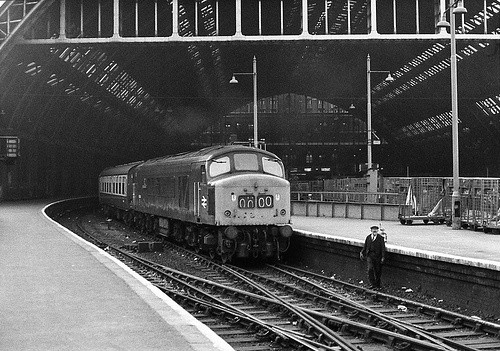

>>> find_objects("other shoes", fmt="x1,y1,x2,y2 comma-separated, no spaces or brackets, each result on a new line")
376,287,382,291
369,285,374,289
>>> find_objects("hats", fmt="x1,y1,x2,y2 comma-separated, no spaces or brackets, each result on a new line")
371,226,379,229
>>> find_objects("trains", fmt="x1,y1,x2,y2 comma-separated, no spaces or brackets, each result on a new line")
97,139,293,265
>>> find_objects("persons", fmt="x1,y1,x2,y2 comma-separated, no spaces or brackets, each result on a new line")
360,226,386,290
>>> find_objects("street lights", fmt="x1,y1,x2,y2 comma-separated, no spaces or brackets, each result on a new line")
436,0,467,230
365,53,395,203
229,56,259,147
